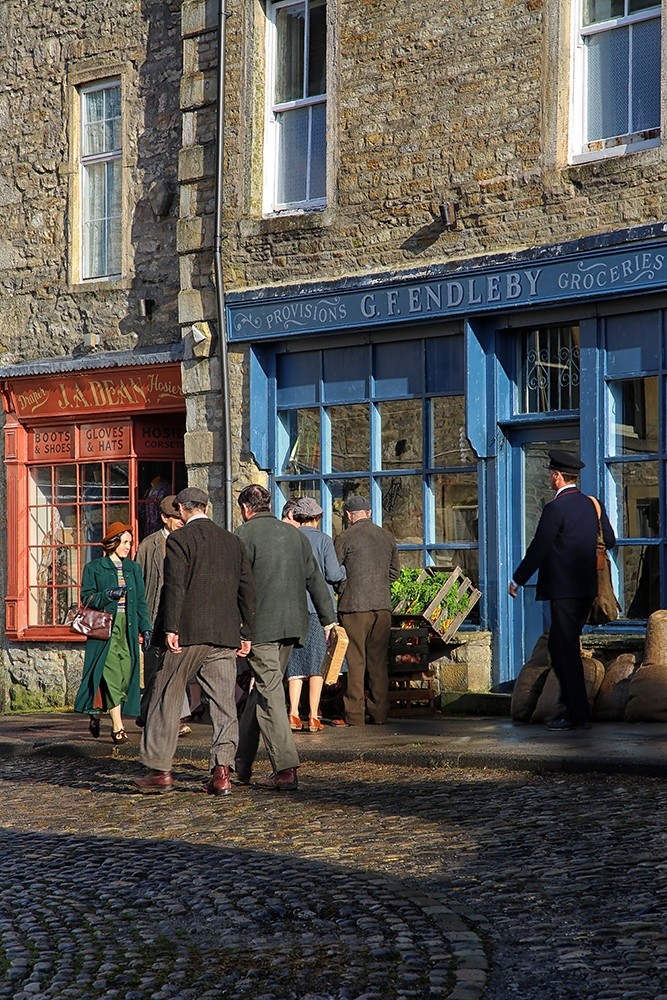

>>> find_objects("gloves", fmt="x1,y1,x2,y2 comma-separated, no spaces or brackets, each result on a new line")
142,630,152,649
109,587,125,599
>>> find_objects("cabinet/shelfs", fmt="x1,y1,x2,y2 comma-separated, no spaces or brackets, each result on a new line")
384,626,437,714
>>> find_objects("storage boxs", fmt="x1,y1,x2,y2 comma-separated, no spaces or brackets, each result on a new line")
390,564,483,646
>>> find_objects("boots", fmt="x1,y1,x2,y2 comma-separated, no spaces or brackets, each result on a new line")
229,758,252,786
255,767,298,790
128,771,172,793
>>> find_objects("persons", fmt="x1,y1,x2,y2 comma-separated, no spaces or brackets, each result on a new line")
82,470,125,560
508,448,616,733
248,496,400,733
73,521,154,744
132,486,258,793
627,501,660,619
229,484,339,792
38,508,68,625
135,494,193,738
138,476,172,542
181,677,248,723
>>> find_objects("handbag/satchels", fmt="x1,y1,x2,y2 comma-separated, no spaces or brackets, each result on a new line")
138,488,151,523
71,595,114,639
588,495,624,621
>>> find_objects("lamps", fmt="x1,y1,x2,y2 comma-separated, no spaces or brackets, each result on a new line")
440,200,458,227
137,297,157,319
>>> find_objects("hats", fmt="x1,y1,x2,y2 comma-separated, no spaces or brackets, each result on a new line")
173,488,208,506
543,450,585,476
160,495,184,516
100,522,133,543
294,496,323,516
342,496,371,512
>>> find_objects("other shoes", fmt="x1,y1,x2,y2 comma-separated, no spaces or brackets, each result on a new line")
111,730,131,744
331,715,384,729
202,765,232,795
89,714,102,738
546,713,592,730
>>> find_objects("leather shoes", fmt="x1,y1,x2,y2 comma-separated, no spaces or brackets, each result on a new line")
289,715,304,730
308,718,325,731
